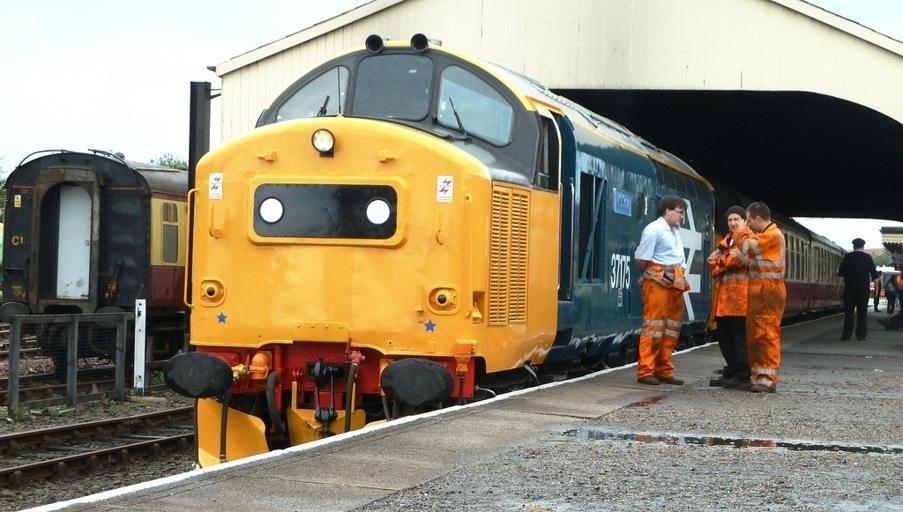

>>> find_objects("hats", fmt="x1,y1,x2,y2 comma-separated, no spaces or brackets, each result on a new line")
725,205,747,219
852,238,865,247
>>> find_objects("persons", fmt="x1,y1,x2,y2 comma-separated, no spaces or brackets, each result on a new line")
885,272,903,313
874,271,882,312
633,196,691,386
837,238,877,341
708,206,754,389
732,202,788,392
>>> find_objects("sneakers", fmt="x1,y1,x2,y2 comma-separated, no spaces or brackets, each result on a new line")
637,375,660,385
877,318,889,328
709,376,776,393
663,376,684,385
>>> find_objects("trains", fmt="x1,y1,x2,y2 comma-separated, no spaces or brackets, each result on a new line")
164,33,846,470
0,148,189,374
